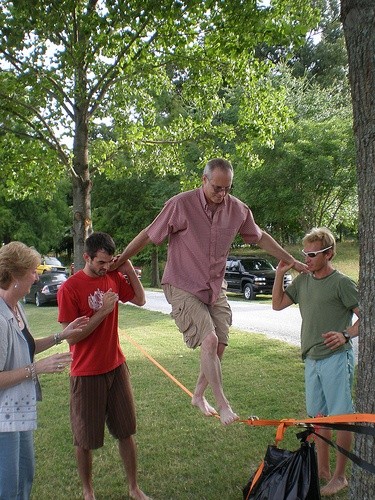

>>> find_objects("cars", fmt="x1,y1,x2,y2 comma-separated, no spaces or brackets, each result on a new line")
22,256,69,307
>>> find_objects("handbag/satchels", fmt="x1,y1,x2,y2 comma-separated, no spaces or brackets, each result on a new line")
242,422,322,500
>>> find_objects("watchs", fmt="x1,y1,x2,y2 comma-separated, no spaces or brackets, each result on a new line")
342,330,349,344
54,333,62,345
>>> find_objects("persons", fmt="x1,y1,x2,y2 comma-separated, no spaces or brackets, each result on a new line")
272,227,361,497
108,158,309,426
0,242,90,500
58,234,153,500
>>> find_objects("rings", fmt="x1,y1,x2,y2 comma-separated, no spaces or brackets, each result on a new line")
77,322,79,325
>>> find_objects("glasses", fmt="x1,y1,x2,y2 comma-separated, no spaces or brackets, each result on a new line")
204,174,231,192
301,246,333,257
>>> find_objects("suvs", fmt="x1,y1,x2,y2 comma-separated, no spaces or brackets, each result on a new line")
225,255,292,300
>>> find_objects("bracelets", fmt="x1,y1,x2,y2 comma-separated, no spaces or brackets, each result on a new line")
25,363,36,381
